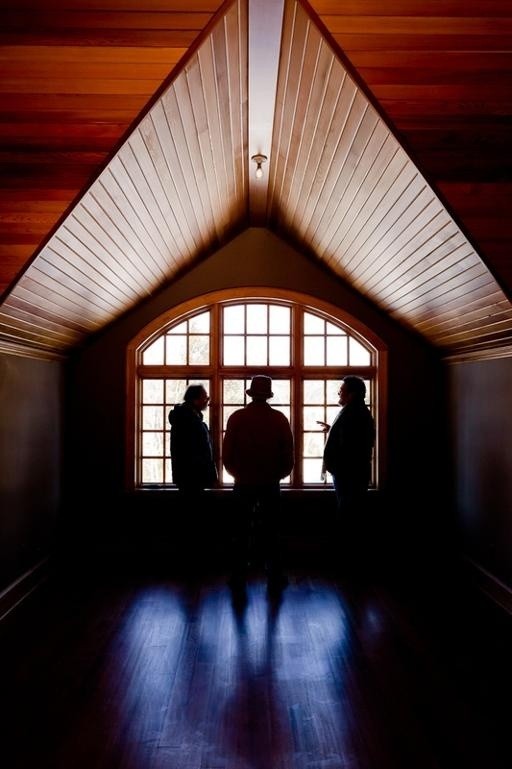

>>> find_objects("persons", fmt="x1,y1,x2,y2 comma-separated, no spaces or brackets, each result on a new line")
314,373,377,581
219,375,298,597
166,383,223,585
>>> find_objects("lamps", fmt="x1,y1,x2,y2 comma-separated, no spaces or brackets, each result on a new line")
251,152,267,180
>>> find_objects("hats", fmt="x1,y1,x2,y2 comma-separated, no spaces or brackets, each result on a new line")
246,375,273,398
